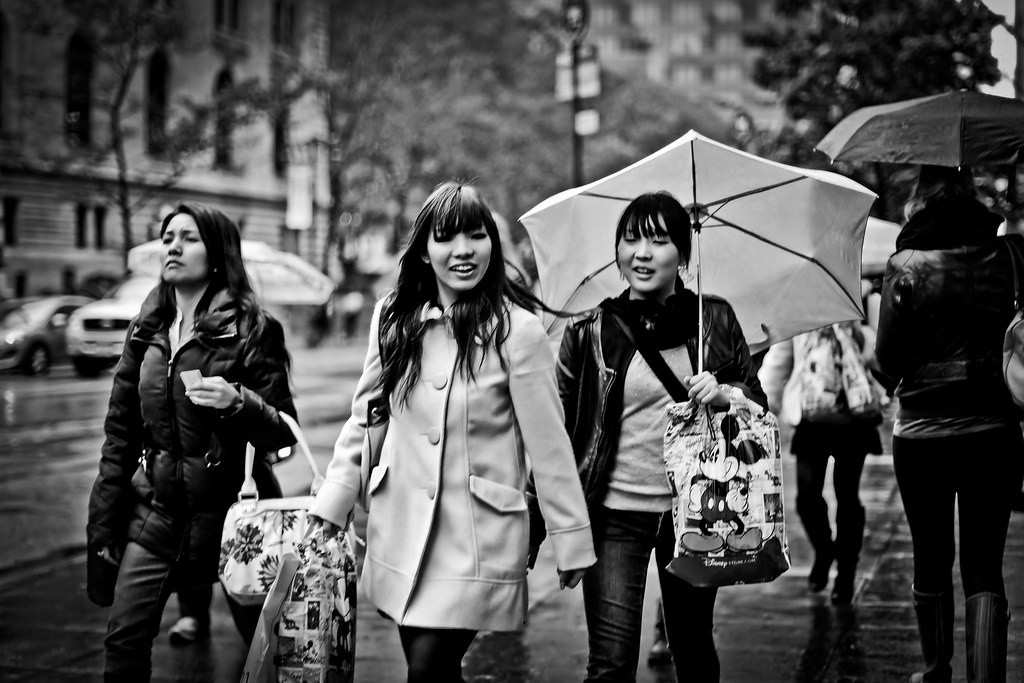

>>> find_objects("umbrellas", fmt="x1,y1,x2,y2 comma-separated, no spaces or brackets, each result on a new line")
861,215,904,278
516,128,881,366
812,88,1024,173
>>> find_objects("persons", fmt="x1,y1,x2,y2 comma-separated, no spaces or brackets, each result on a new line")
341,283,364,340
306,297,337,349
867,164,1024,683
166,555,266,652
304,176,599,683
647,596,673,667
84,202,301,683
756,278,895,609
522,189,772,683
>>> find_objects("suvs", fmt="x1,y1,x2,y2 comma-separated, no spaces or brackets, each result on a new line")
65,253,338,376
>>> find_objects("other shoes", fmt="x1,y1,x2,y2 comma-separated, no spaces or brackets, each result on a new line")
168,617,211,646
647,625,670,666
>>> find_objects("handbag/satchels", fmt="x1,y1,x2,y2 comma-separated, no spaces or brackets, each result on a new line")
217,412,366,606
357,292,398,513
1001,233,1024,405
663,382,793,587
239,524,357,683
802,324,892,425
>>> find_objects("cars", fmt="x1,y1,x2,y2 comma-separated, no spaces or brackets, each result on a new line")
0,295,93,375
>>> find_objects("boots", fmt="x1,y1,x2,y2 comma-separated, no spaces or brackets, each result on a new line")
964,592,1011,683
910,583,955,683
800,505,836,590
831,505,866,603
